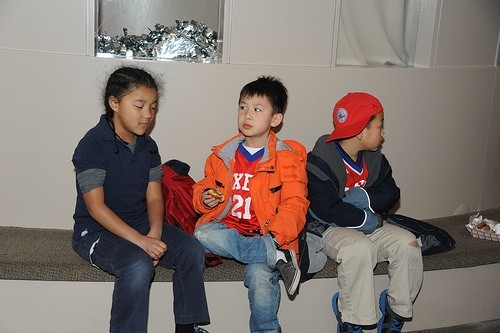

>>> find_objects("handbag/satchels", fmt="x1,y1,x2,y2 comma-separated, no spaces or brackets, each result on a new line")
387,213,456,256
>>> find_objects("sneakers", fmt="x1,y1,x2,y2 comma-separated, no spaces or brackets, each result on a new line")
377,289,405,333
275,248,301,295
331,291,364,333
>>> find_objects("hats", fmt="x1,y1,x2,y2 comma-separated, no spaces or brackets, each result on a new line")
325,92,384,141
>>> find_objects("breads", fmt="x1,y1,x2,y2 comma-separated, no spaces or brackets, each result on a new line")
475,222,490,230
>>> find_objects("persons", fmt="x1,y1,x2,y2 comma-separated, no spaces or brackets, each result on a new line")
305,91,424,333
71,66,205,333
191,76,311,333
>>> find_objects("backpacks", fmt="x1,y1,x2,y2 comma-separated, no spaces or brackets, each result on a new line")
162,159,223,267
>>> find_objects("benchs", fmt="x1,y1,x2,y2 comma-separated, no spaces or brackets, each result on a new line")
1,205,500,333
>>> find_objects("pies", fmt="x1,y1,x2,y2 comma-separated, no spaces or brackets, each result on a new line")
206,189,224,202
